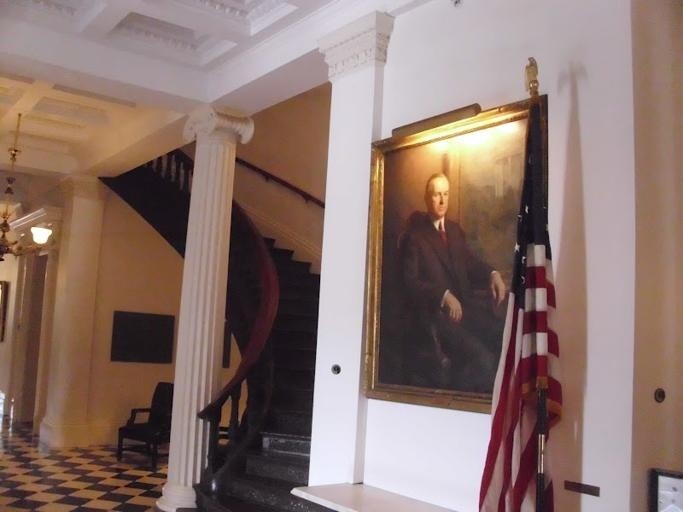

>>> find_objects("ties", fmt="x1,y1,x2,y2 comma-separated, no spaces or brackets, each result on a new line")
439,222,448,249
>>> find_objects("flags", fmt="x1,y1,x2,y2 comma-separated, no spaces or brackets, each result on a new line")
479,100,563,512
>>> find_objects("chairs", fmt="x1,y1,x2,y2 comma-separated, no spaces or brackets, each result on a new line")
117,382,174,471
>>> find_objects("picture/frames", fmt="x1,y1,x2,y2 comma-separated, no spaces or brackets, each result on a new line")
360,95,548,414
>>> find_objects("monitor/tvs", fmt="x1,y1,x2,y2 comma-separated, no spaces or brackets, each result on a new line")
111,310,176,364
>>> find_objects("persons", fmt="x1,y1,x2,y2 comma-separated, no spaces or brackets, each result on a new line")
397,170,509,394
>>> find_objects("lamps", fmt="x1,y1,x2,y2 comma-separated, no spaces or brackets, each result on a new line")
0,113,53,258
